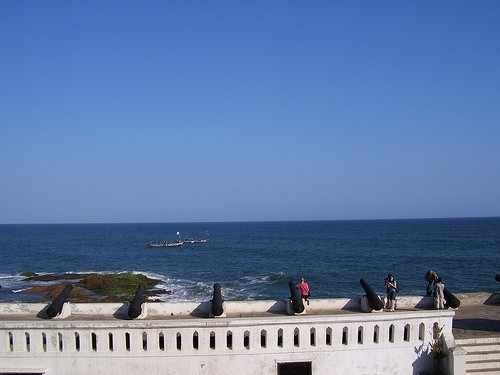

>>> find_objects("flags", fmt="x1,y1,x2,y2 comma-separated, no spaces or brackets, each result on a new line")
177,232,179,235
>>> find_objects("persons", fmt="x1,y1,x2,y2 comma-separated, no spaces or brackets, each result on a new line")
296,277,310,305
384,273,397,311
426,277,446,309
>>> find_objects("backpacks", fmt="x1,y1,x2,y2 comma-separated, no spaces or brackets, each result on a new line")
393,281,399,293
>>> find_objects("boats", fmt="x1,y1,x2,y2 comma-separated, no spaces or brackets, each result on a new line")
145,231,208,247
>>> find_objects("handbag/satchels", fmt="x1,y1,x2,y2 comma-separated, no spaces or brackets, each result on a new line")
308,294,311,297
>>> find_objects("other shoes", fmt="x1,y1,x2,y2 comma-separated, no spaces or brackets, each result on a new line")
389,309,394,312
307,301,309,305
